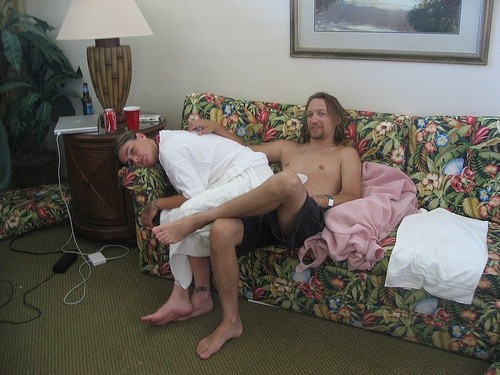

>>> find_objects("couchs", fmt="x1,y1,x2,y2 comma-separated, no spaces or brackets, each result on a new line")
123,92,500,362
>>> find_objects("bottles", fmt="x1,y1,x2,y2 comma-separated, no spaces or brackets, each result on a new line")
82,83,93,115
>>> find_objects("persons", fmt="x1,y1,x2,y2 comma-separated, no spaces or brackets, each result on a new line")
113,128,276,326
152,92,363,359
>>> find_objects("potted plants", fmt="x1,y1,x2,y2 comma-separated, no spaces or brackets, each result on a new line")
0,0,83,190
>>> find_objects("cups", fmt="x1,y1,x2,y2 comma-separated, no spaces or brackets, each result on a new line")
124,106,141,131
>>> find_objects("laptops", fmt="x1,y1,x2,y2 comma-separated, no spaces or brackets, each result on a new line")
53,114,99,135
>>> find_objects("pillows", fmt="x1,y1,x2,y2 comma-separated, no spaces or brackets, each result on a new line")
0,183,73,240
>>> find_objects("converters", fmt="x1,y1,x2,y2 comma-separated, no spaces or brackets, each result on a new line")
51,250,78,274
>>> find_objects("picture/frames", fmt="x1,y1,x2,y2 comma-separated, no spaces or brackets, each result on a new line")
290,0,494,66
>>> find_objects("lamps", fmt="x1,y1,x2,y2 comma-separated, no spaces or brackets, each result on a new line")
56,0,154,122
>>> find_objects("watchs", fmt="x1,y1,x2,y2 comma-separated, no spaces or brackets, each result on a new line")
325,194,334,210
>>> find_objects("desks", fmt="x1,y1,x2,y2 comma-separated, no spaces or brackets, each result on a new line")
61,110,168,248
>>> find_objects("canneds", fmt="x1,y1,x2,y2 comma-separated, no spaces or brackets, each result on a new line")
103,108,117,132
